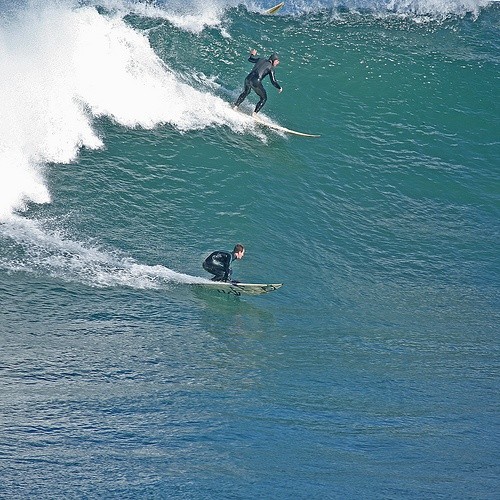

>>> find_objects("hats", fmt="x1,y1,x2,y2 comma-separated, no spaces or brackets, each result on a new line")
269,53,278,66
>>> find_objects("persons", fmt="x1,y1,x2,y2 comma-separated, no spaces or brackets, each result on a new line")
202,244,245,282
233,50,283,119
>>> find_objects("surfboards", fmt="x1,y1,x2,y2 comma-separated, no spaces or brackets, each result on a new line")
238,112,321,138
201,283,284,295
